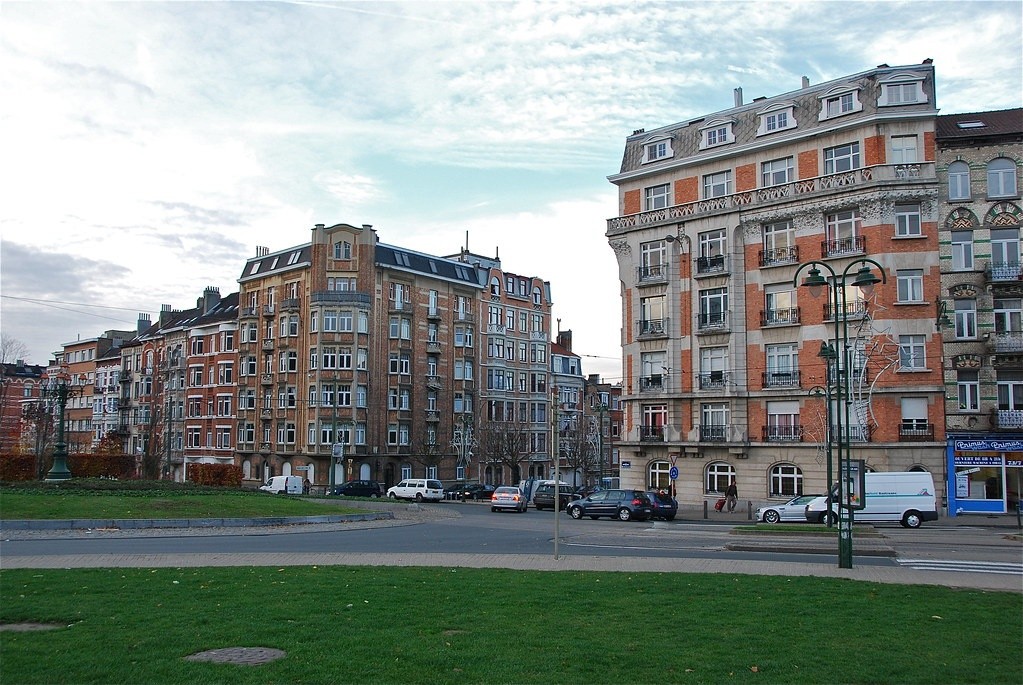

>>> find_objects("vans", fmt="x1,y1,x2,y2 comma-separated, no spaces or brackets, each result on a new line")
804,472,939,529
260,476,303,495
329,480,382,498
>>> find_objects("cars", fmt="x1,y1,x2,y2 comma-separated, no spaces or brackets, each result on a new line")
755,494,823,524
457,483,496,500
576,485,605,498
491,486,528,513
443,484,471,500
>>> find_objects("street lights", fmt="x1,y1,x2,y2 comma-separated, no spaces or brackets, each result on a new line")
161,368,175,478
592,401,608,488
41,359,89,482
808,341,845,528
792,258,888,568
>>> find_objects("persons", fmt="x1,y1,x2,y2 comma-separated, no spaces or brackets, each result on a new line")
667,479,676,499
725,481,739,513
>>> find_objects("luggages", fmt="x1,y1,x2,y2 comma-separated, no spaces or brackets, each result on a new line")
714,498,727,512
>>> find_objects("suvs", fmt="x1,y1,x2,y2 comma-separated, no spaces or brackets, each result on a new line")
646,491,677,522
386,478,445,503
566,489,653,521
533,484,583,511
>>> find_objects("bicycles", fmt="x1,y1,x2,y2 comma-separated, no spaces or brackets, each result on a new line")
302,485,317,495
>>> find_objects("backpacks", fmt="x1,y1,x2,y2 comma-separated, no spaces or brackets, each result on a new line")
724,485,736,498
